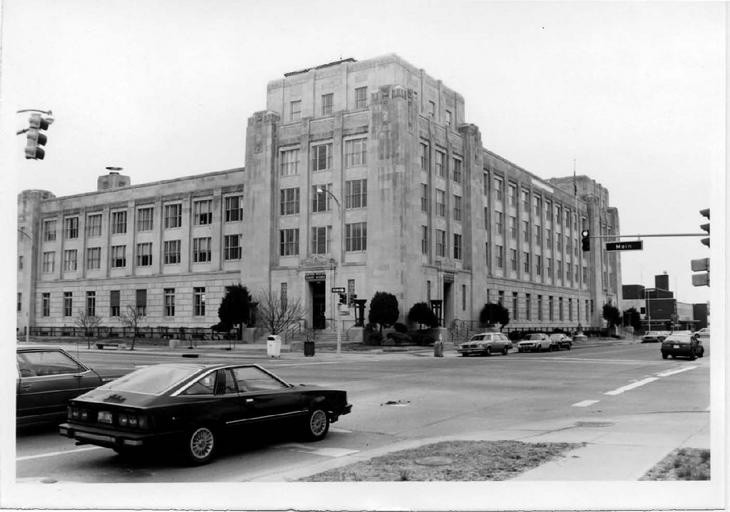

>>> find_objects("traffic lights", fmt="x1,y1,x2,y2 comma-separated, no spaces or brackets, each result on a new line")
582,230,590,251
700,208,710,247
24,113,48,160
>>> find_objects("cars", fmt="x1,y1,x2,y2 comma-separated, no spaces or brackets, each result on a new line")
457,332,513,356
642,328,710,359
58,364,351,463
16,348,137,422
517,334,573,352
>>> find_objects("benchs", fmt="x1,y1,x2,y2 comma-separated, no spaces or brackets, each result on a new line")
95,343,128,350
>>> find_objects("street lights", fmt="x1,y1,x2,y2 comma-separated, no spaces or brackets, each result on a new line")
317,188,341,353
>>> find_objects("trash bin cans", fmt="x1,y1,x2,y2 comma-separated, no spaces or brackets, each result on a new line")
267,335,281,356
304,341,314,357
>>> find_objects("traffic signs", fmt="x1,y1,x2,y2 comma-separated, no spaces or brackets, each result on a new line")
332,288,344,292
